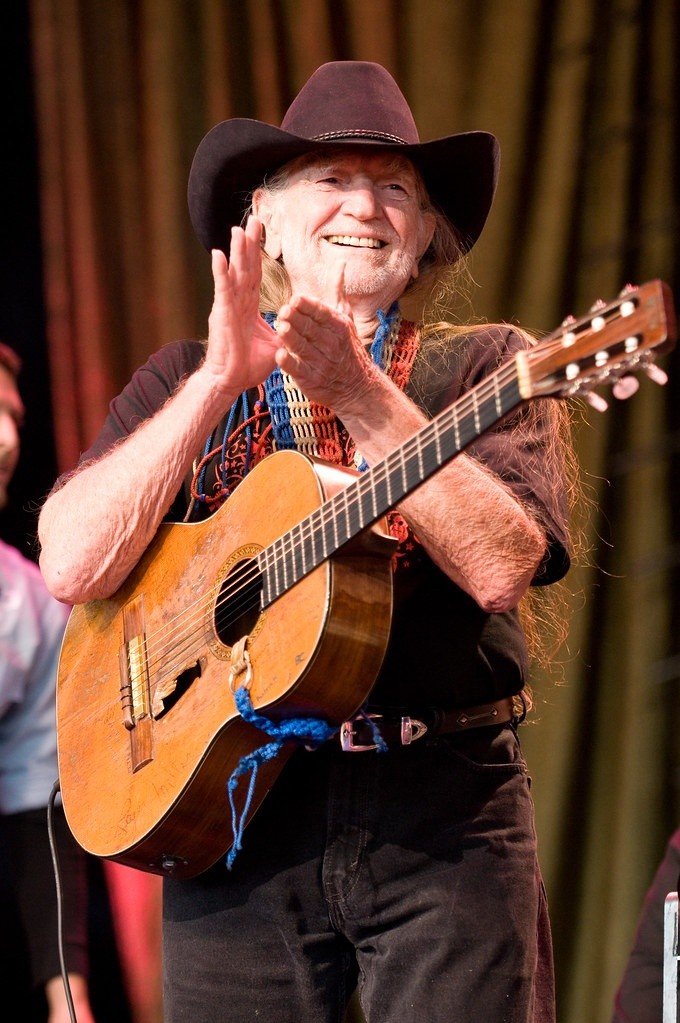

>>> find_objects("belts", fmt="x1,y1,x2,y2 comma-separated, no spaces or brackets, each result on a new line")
286,682,535,759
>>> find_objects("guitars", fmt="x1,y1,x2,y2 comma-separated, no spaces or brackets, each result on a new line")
54,277,676,886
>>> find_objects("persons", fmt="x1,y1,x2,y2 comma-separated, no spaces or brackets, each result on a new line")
612,829,680,1022
37,60,628,1023
0,346,95,1022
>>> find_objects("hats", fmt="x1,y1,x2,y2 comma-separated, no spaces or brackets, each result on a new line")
188,59,498,295
